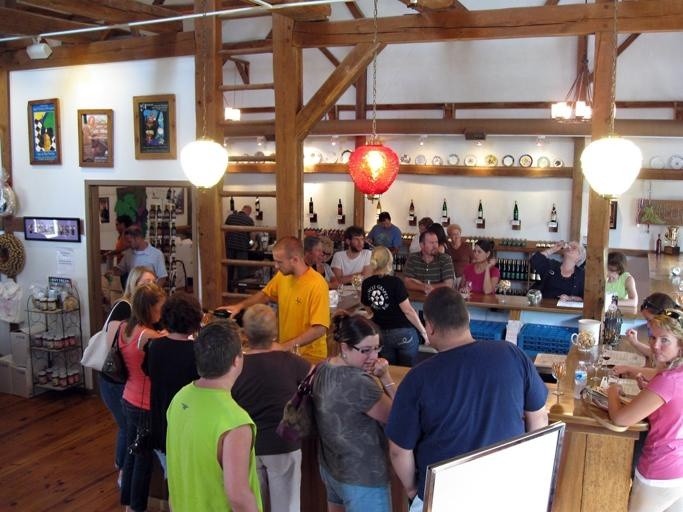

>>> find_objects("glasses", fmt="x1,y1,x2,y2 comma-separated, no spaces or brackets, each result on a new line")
642,298,661,310
351,345,383,354
659,309,681,323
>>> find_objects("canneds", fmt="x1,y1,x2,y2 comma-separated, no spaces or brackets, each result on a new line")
40,297,56,311
32,331,80,389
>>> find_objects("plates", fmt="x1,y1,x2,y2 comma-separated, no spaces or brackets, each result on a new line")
308,149,350,166
518,153,532,168
536,156,549,167
501,155,514,167
484,153,498,166
400,151,477,167
650,155,682,171
553,158,562,168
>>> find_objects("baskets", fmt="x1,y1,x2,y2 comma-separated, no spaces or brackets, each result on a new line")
469,319,507,341
517,322,578,355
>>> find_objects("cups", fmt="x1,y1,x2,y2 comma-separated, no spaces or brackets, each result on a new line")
570,332,591,352
328,289,340,308
337,284,344,302
577,319,600,345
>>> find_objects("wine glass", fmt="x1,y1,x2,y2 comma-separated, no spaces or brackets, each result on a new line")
602,343,612,370
550,357,566,395
457,281,473,298
422,280,430,296
586,347,601,381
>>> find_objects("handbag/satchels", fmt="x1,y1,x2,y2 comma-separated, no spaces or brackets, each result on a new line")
276,381,316,444
127,431,152,458
80,330,112,372
101,337,130,384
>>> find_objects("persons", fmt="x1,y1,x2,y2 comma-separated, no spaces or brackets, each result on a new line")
95,264,158,489
613,293,683,380
139,291,203,480
384,286,548,511
607,307,682,511
114,206,639,309
118,280,169,511
316,310,398,512
231,303,316,511
360,245,428,365
82,116,100,160
165,320,263,512
215,234,331,359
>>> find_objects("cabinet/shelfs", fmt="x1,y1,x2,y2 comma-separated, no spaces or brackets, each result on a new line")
302,229,561,296
26,286,84,398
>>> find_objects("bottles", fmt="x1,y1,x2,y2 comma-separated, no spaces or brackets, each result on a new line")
202,307,242,327
477,200,482,219
336,199,342,216
550,203,556,223
513,201,518,221
254,195,260,212
392,253,408,271
494,256,541,297
308,197,314,213
147,186,176,291
304,227,558,248
655,234,661,255
573,360,587,402
603,293,622,348
376,200,382,215
228,196,234,210
408,199,414,217
442,198,447,217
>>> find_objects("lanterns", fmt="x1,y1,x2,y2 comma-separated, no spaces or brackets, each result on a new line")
178,138,228,196
347,145,399,202
579,138,643,201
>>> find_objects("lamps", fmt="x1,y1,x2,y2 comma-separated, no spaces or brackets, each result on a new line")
551,34,597,124
220,51,243,123
25,34,52,62
179,13,228,193
574,0,644,205
348,0,399,204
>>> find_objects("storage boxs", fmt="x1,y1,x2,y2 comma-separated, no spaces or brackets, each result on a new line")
0,321,50,398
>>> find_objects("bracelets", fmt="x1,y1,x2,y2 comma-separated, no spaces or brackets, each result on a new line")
382,381,395,388
419,327,425,335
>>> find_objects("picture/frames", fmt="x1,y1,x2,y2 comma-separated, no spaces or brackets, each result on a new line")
26,96,61,166
608,198,619,230
20,215,81,245
132,92,177,161
76,107,114,169
